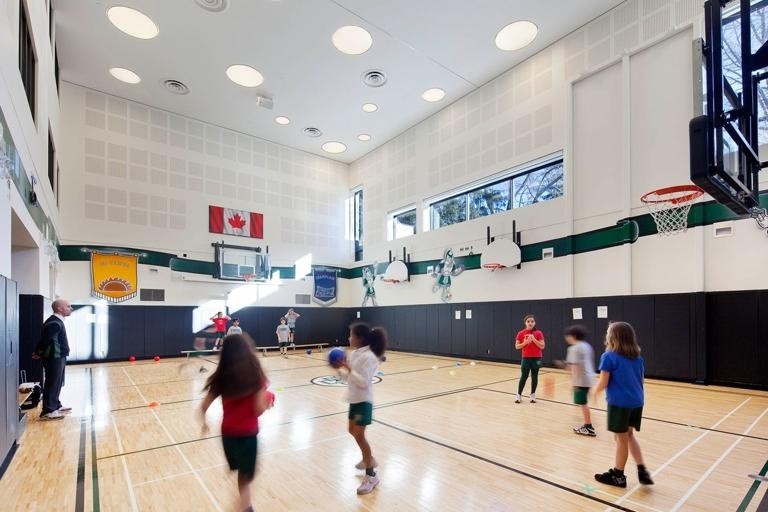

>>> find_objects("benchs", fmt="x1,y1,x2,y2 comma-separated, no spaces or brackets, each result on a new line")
19,382,40,415
180,343,329,361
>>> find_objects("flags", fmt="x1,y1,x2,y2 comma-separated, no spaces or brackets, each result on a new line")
311,269,337,307
208,205,264,240
90,251,138,304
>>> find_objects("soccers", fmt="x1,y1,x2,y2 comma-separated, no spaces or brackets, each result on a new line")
128,356,135,362
327,350,346,368
306,349,311,354
153,356,160,362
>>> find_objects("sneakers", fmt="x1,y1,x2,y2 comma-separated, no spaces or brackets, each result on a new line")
355,456,378,469
39,410,64,421
357,472,380,494
58,406,72,413
514,392,654,488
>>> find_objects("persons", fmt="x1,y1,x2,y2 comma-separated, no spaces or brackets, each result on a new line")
361,259,380,307
209,311,231,351
430,248,465,302
513,314,546,404
197,333,272,512
31,298,74,421
227,317,242,336
552,324,598,438
590,322,656,489
284,308,300,347
334,322,389,496
276,317,291,359
